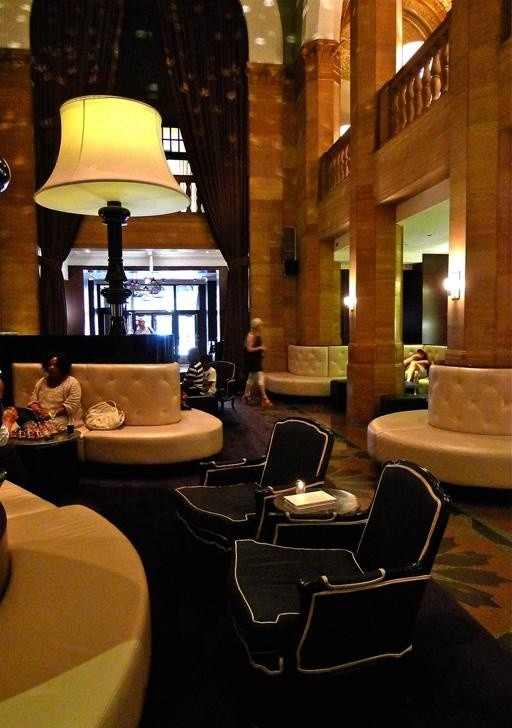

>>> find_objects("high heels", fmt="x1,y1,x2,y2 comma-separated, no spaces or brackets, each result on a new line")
261,397,274,407
243,394,255,405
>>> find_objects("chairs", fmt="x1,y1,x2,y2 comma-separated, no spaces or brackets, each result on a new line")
208,361,238,415
175,416,333,551
223,460,450,675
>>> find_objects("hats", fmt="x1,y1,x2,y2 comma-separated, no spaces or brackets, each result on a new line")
251,318,263,327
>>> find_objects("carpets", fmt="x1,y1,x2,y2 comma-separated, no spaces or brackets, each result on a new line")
0,407,512,728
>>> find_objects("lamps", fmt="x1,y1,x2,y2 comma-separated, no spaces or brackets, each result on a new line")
126,272,166,301
32,94,191,335
442,271,461,300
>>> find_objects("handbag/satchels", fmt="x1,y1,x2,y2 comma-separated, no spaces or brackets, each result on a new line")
86,400,124,429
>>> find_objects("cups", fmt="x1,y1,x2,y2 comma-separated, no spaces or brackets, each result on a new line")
11,414,76,440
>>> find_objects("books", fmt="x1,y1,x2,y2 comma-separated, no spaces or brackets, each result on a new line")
283,491,337,516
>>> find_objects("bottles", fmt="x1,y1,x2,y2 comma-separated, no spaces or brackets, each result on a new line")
413,370,419,383
413,385,418,396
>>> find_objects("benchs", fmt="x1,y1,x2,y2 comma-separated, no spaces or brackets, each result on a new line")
0,478,152,728
12,362,224,464
367,361,512,488
264,344,449,396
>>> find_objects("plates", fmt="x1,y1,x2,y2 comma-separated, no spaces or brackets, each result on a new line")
272,488,360,517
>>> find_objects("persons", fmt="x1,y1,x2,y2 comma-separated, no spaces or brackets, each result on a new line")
0,380,19,447
135,318,156,335
26,352,83,432
180,347,203,396
403,349,430,381
200,355,218,395
242,318,274,408
